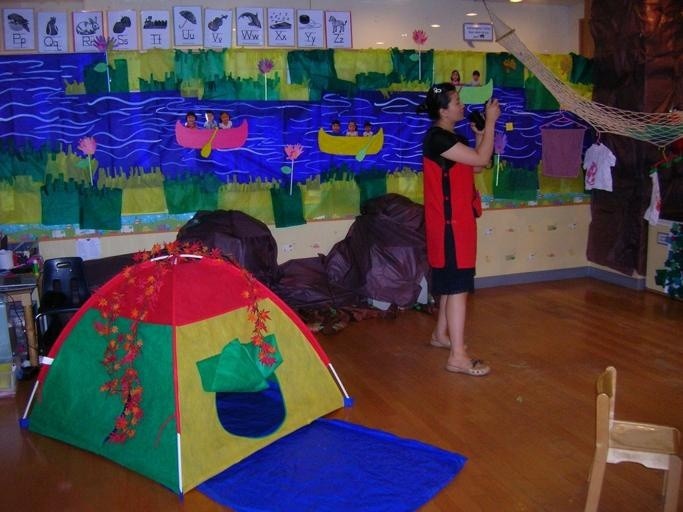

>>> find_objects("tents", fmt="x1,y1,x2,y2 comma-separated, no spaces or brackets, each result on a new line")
18,240,350,503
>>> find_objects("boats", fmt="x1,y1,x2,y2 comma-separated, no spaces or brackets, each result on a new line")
316,126,385,157
174,118,249,151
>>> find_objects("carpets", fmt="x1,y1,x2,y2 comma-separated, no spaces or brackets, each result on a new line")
197,418,467,512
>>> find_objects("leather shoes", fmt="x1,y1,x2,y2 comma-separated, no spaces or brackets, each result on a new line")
446,357,491,375
431,334,469,351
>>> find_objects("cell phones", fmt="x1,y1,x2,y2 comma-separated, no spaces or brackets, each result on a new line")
468,110,486,131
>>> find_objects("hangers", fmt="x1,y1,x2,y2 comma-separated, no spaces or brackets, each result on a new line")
593,131,601,144
648,144,683,173
539,108,587,132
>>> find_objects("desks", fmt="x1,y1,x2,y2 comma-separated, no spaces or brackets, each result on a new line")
0,271,44,367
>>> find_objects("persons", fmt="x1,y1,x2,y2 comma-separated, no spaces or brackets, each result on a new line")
361,121,373,137
328,121,344,136
412,81,503,378
448,70,462,86
218,112,233,129
202,110,218,129
346,121,359,137
183,111,198,129
469,70,480,86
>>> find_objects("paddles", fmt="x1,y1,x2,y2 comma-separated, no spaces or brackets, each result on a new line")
356,135,376,161
200,127,219,158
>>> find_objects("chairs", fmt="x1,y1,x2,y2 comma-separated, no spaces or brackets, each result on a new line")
35,257,91,356
584,367,683,512
